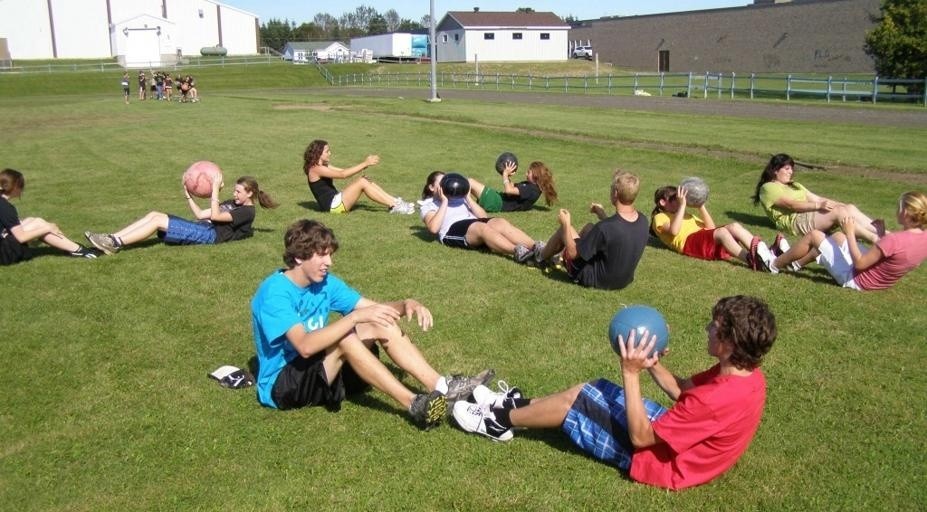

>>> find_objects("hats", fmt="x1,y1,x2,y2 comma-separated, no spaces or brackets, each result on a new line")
208,365,255,390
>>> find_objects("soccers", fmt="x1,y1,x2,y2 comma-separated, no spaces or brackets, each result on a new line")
439,173,469,199
678,176,711,207
494,152,517,176
184,160,223,198
609,305,668,357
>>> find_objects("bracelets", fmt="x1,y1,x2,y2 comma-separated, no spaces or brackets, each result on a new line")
503,179,510,184
184,195,192,199
211,198,220,202
815,202,818,209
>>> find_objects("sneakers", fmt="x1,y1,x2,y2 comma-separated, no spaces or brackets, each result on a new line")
767,235,782,256
453,400,515,441
779,239,797,273
71,242,99,259
535,241,546,262
84,231,119,256
746,236,760,270
473,380,522,409
757,241,779,274
410,390,448,431
389,197,415,215
444,369,496,415
513,245,534,263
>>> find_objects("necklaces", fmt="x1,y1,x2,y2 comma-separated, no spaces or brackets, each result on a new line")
615,205,637,217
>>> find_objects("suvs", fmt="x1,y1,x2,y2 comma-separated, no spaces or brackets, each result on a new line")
573,45,593,59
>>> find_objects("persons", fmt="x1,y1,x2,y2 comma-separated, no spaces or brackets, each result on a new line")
650,186,784,272
751,154,891,244
122,72,130,104
453,294,778,490
250,218,495,432
757,190,927,292
139,66,201,103
303,140,416,215
417,160,560,212
416,171,547,264
535,168,649,291
85,176,280,256
0,168,100,265
